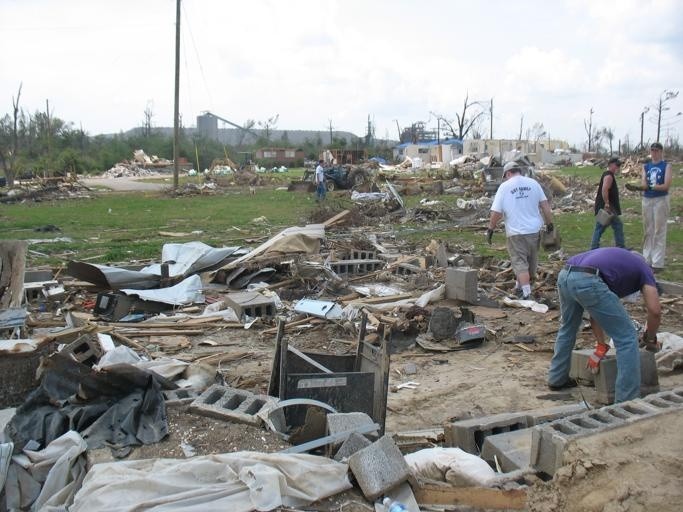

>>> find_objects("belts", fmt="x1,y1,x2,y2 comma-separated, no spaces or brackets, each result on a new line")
565,264,596,274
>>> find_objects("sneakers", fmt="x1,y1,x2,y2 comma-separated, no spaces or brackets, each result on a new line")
510,287,522,298
550,378,576,391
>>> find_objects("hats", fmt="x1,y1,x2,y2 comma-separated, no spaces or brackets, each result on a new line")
651,142,663,149
502,161,521,176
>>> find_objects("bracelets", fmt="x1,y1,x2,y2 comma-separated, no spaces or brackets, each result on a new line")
643,331,657,344
649,185,655,191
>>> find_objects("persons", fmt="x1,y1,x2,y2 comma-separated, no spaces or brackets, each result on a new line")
486,161,554,300
547,246,661,404
625,143,673,275
591,158,634,250
315,159,328,202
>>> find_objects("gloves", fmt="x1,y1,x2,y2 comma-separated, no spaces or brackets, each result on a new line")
585,342,610,375
486,229,493,244
640,330,660,352
